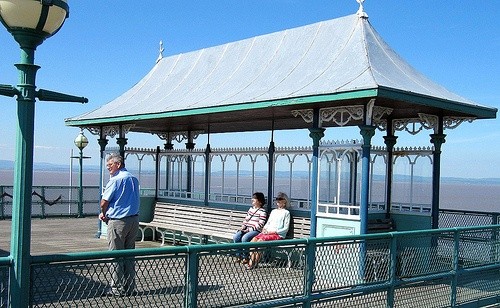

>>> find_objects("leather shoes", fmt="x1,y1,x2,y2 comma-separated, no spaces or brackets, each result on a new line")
236,257,241,263
242,259,248,264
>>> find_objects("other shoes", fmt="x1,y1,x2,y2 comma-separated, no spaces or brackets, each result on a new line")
104,286,137,296
246,262,258,269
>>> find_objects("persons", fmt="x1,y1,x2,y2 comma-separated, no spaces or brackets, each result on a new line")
98,152,141,297
243,192,290,270
233,192,267,265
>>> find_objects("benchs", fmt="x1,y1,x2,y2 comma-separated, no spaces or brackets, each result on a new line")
135,202,311,268
365,218,416,281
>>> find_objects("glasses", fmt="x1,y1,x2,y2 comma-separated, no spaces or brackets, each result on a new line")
276,197,285,201
106,163,117,167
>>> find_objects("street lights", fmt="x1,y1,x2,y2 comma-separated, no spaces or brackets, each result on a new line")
70,129,92,218
0,0,89,308
